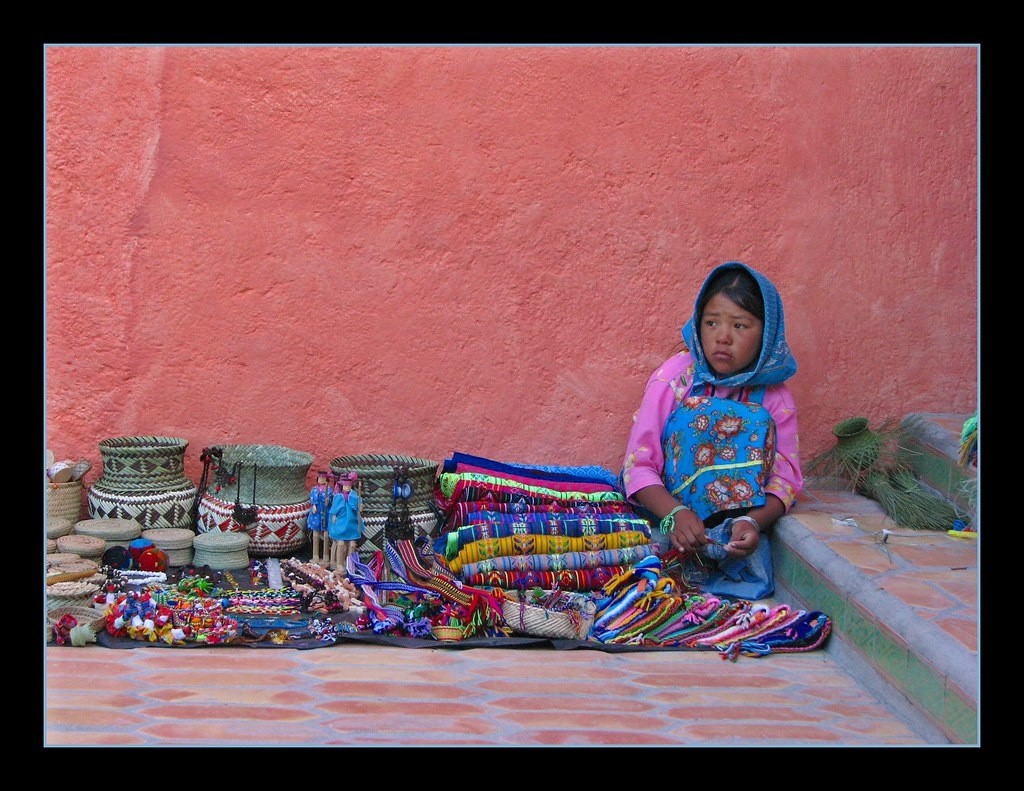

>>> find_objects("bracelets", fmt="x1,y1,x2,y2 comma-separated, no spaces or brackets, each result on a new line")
659,505,689,535
732,516,760,533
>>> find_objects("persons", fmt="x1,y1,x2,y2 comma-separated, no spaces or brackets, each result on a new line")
620,261,805,600
307,471,363,571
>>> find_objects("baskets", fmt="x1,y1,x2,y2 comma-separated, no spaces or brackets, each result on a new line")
430,625,465,642
85,435,198,530
46,559,107,643
328,452,438,565
46,478,83,527
196,445,314,557
502,588,596,640
